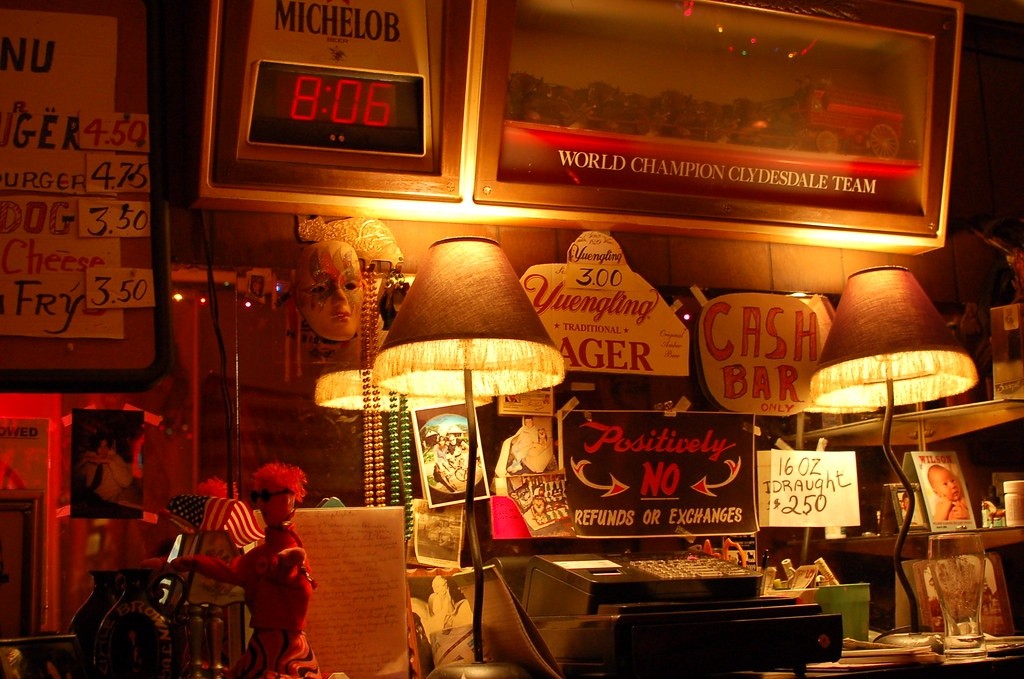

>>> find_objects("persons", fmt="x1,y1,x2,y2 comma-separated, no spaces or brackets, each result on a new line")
433,434,468,492
429,575,454,616
928,464,972,524
76,435,130,499
429,520,453,547
174,459,322,679
494,416,538,476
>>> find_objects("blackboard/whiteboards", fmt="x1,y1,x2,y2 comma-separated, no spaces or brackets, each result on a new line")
558,410,759,536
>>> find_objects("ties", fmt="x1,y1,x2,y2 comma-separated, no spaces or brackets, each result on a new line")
90,464,103,491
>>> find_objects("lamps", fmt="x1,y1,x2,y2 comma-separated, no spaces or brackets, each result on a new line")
314,275,493,505
375,238,564,679
810,263,977,650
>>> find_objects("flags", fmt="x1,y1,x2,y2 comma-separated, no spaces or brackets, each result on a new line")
159,493,267,550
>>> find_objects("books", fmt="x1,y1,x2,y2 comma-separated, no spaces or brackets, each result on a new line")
839,643,945,664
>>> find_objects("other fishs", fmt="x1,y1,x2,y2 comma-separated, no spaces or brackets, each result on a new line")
295,215,404,265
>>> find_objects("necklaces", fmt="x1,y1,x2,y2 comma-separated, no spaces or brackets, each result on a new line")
390,391,414,541
362,269,387,509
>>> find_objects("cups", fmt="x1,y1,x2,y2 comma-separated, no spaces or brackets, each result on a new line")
817,583,870,642
927,533,987,662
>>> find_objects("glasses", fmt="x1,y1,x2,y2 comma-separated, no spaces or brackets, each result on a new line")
250,488,290,503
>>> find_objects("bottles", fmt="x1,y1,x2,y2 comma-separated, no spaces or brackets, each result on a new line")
1003,479,1024,527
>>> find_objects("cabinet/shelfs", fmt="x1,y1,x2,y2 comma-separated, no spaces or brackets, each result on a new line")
814,398,1024,560
